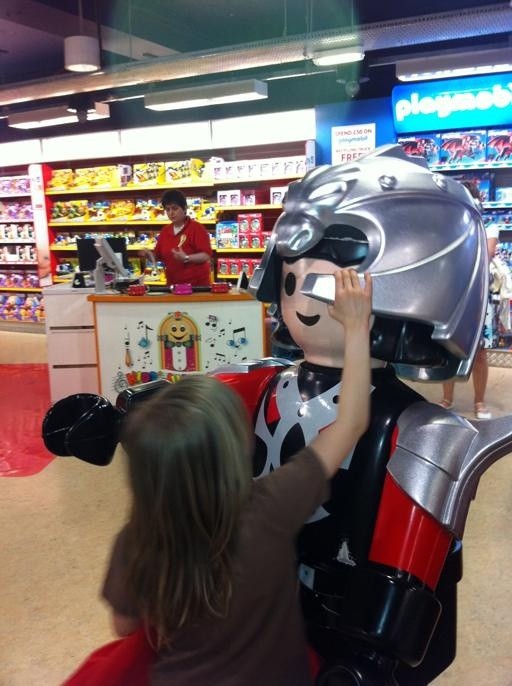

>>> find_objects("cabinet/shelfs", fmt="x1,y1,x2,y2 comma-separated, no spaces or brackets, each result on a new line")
0,140,314,334
43,283,100,406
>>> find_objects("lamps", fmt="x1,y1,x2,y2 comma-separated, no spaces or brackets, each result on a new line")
60,1,102,73
395,47,512,82
143,80,269,112
312,46,366,68
7,102,111,130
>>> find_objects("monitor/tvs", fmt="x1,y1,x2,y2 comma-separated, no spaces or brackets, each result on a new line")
76,238,127,269
91,236,129,295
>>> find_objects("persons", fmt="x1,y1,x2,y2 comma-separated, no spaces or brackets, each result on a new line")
434,182,499,420
100,269,374,686
137,189,212,287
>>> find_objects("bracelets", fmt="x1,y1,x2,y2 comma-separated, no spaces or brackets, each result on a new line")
184,255,189,264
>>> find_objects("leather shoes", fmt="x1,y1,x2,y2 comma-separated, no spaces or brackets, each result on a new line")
437,399,456,410
474,403,492,420
